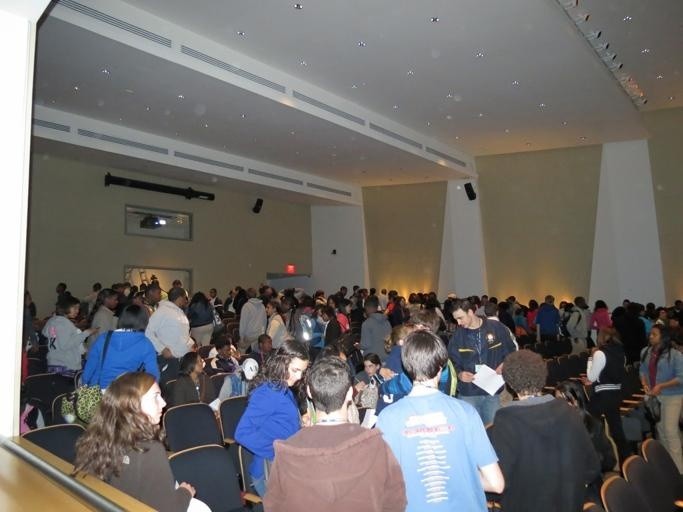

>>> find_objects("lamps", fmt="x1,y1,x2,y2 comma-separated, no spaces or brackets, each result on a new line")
551,0,649,113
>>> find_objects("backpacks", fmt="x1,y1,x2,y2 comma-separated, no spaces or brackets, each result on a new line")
559,309,582,338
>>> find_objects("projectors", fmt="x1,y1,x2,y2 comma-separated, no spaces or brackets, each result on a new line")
140,218,162,229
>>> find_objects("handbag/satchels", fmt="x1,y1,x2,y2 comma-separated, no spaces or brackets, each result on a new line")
353,383,378,409
642,394,662,423
60,383,103,428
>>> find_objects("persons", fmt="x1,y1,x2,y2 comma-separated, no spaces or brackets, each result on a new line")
23,278,682,508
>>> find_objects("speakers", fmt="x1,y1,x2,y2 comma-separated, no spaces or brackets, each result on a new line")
252,198,263,214
464,183,476,200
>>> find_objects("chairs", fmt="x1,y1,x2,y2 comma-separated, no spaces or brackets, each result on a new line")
601,476,647,511
622,454,682,512
506,334,660,450
18,310,372,512
640,438,682,498
